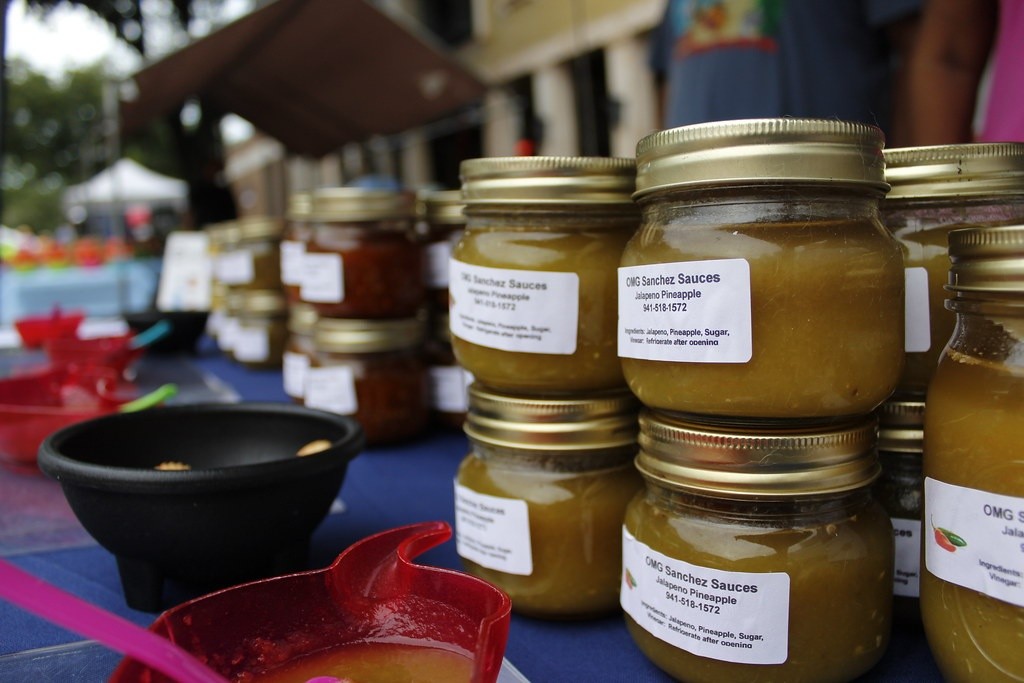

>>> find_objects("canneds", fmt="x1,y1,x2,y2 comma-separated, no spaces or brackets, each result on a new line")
202,119,1024,683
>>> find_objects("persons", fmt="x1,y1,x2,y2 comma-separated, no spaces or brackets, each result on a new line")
642,0,1024,225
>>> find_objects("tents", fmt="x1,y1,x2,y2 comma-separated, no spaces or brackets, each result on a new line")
63,156,187,224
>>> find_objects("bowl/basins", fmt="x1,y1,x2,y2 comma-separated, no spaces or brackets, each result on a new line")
111,522,512,683
37,402,364,614
0,315,141,475
